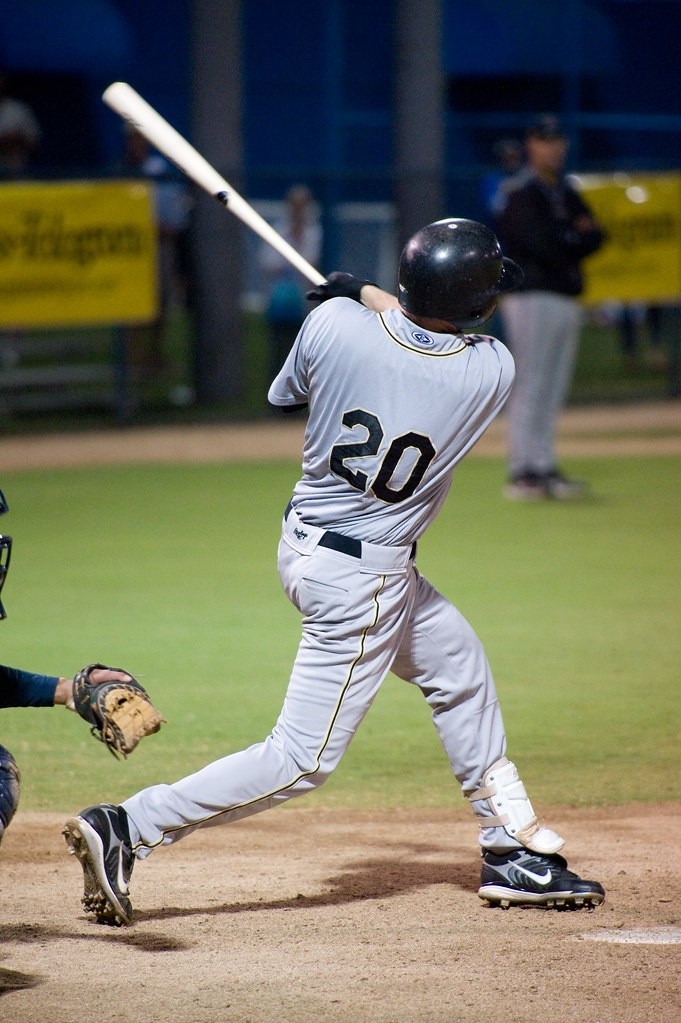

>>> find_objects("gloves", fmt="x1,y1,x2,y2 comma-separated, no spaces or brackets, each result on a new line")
306,271,382,309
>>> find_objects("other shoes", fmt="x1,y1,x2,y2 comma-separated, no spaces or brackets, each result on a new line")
542,468,580,502
502,473,545,502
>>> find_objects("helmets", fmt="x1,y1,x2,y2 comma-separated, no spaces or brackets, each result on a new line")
398,217,523,328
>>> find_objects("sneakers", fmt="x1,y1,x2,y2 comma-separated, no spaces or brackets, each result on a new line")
61,802,136,926
478,847,605,911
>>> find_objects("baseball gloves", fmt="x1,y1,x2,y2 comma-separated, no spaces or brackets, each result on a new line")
70,663,165,759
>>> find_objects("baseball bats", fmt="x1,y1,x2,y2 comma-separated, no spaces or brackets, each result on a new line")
103,82,326,285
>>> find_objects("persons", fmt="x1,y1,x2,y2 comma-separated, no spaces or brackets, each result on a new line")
481,143,524,216
0,491,166,841
259,186,324,368
60,218,606,926
495,116,612,501
